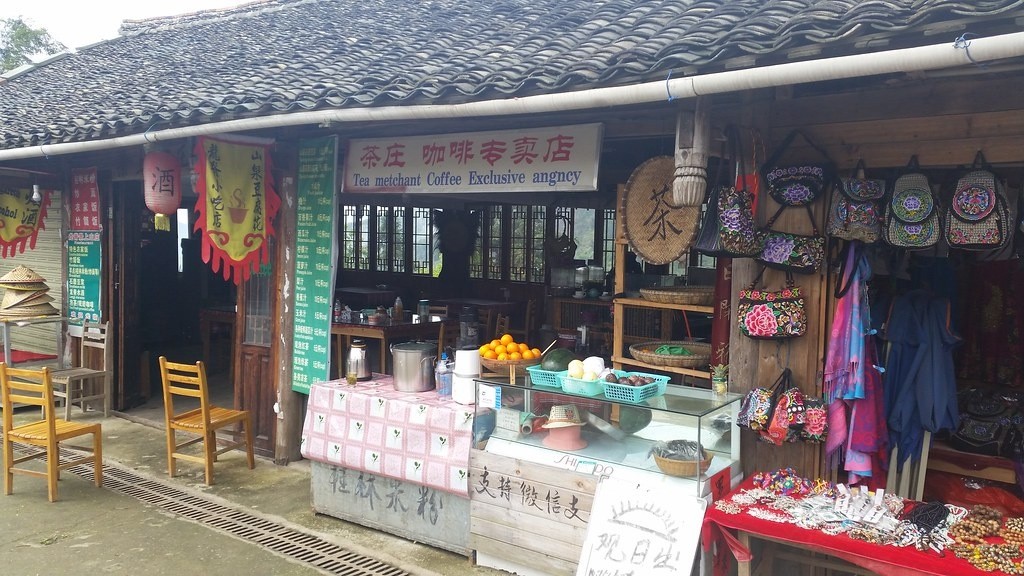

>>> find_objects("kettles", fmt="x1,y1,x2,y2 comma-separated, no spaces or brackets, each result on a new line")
345,339,372,381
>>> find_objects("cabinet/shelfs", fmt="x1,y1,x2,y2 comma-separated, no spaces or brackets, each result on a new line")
0,317,68,413
300,371,747,576
609,184,715,422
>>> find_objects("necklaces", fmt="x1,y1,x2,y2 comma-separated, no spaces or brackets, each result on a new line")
902,500,968,552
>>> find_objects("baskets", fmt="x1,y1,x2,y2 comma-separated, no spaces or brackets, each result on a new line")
638,284,716,305
629,338,712,367
653,449,714,477
621,155,702,266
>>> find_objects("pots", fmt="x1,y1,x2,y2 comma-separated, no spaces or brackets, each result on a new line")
389,340,438,392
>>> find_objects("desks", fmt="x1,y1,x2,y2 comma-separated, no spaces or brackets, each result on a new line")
701,472,1024,576
199,302,235,377
335,286,393,348
449,297,518,315
332,318,460,378
927,441,1017,484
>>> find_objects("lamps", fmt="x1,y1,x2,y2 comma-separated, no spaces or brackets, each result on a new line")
30,158,42,202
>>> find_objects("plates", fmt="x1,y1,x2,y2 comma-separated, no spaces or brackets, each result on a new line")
600,296,612,301
572,295,586,299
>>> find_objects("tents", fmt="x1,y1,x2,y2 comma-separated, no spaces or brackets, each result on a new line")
870,276,962,503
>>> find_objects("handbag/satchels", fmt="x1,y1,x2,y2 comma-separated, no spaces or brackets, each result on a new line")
716,124,831,343
736,368,828,447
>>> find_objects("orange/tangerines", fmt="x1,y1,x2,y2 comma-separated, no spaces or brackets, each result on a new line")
479,334,541,360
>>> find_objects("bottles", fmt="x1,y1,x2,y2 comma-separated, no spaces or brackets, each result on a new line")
367,315,379,325
435,353,453,394
334,298,341,323
417,299,430,323
394,296,403,321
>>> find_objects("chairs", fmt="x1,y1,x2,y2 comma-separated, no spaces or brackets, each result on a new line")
41,318,112,421
477,309,495,341
0,362,102,502
495,313,509,338
159,356,253,484
437,322,459,360
509,297,537,344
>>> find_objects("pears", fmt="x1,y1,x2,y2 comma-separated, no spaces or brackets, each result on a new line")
568,356,610,390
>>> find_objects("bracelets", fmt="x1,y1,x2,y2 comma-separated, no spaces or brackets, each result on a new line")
950,505,1024,575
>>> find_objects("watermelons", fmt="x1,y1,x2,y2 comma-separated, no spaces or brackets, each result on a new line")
541,348,576,384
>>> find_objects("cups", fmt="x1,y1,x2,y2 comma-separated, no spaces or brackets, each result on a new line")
575,291,587,296
345,359,358,386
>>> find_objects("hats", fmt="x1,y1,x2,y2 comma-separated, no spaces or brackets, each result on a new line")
539,404,589,450
0,264,60,323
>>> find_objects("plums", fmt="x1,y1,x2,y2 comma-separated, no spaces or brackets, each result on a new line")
606,372,658,398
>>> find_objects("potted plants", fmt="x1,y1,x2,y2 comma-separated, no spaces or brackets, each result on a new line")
710,363,732,397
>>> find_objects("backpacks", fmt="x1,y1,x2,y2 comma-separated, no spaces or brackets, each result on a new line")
820,150,1020,264
